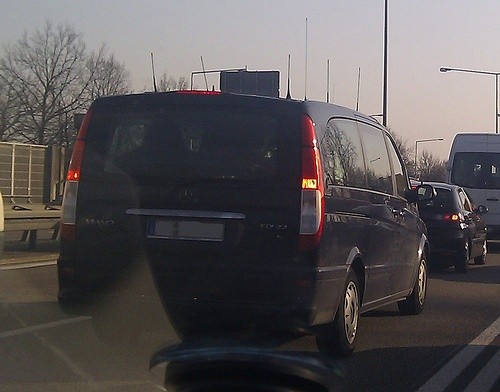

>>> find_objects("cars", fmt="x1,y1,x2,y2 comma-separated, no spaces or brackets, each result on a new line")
404,181,488,273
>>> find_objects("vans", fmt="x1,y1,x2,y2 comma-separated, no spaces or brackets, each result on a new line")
446,132,500,242
56,90,432,358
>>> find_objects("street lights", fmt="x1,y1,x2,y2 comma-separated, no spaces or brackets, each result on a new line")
414,138,444,180
440,67,500,133
